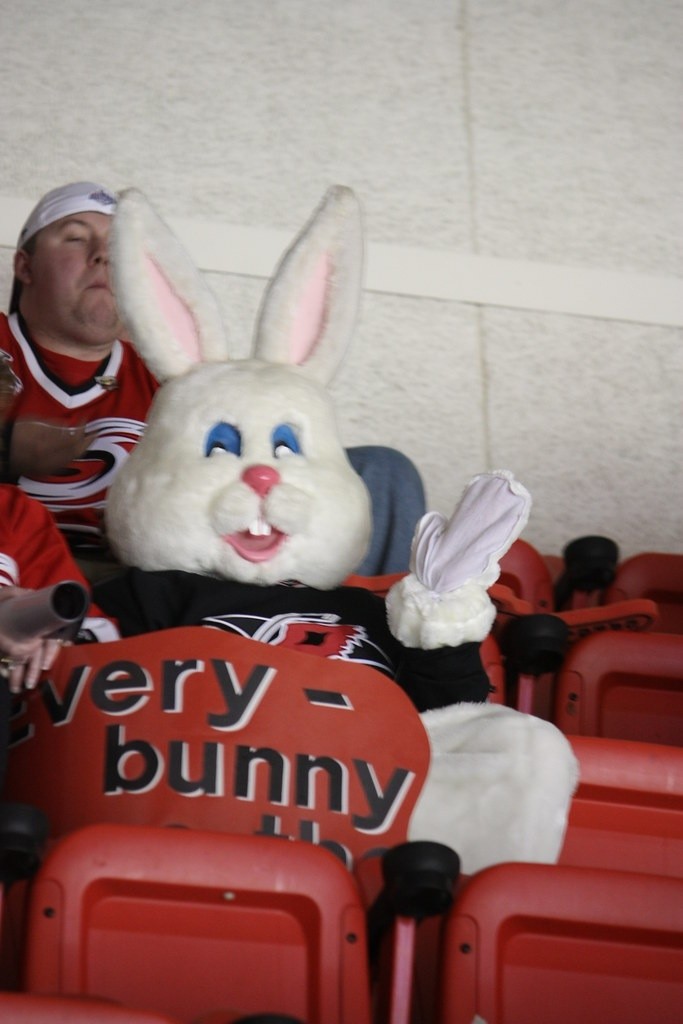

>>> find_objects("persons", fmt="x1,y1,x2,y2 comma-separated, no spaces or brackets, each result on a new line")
1,481,122,697
0,175,168,557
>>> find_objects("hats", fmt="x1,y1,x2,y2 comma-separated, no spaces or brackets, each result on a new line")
10,181,118,315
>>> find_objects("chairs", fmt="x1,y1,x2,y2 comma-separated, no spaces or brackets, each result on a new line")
1,538,683,1024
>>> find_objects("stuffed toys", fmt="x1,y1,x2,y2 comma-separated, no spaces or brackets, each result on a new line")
86,184,581,879
